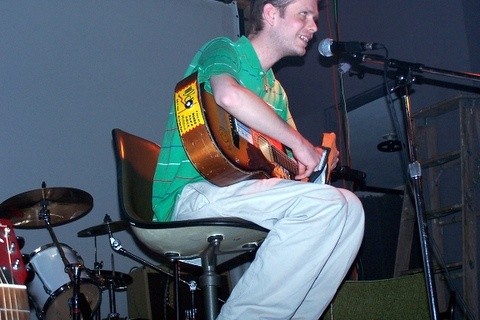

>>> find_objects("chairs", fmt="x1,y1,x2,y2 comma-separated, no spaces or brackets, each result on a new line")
111,126,272,320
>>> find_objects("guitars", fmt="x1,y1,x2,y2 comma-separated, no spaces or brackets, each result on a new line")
174,71,301,187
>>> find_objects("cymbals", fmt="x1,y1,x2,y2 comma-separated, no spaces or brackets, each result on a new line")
0,187,94,231
91,269,133,289
77,221,127,236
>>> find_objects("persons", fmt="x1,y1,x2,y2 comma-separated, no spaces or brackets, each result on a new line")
149,0,365,320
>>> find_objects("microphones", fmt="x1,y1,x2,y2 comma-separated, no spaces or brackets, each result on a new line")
318,38,384,57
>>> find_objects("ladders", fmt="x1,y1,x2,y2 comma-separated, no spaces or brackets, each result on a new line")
409,95,480,320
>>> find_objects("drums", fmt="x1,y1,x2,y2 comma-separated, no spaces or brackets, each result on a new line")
23,242,102,319
